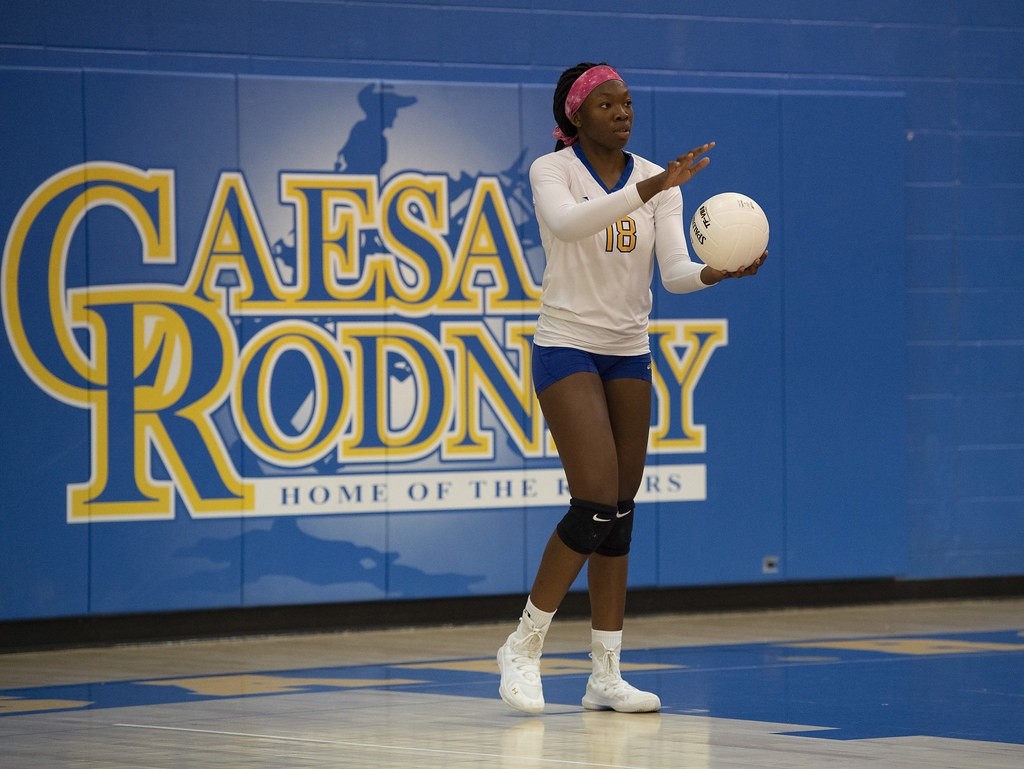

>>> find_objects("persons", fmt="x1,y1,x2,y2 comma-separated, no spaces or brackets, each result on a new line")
496,63,769,714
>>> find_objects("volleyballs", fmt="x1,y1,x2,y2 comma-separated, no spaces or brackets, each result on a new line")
689,191,770,273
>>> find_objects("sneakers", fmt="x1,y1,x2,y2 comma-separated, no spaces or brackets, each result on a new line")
501,722,545,769
497,609,551,714
582,640,661,712
582,711,662,765
